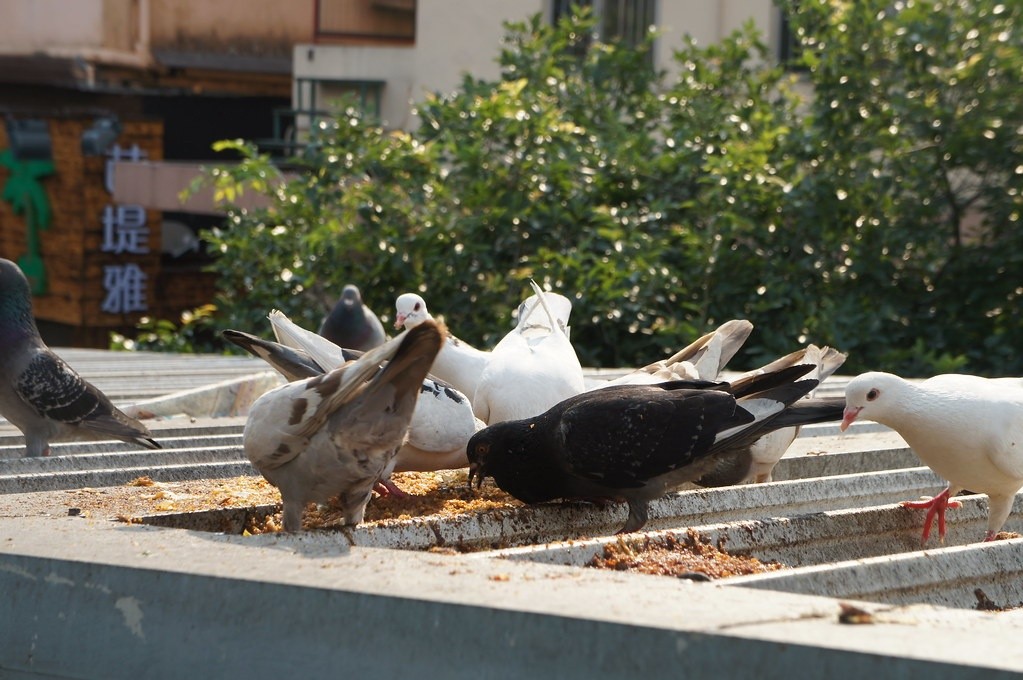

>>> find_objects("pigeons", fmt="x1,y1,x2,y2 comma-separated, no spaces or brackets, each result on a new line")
841,371,1023,547
223,279,847,535
0,258,162,458
